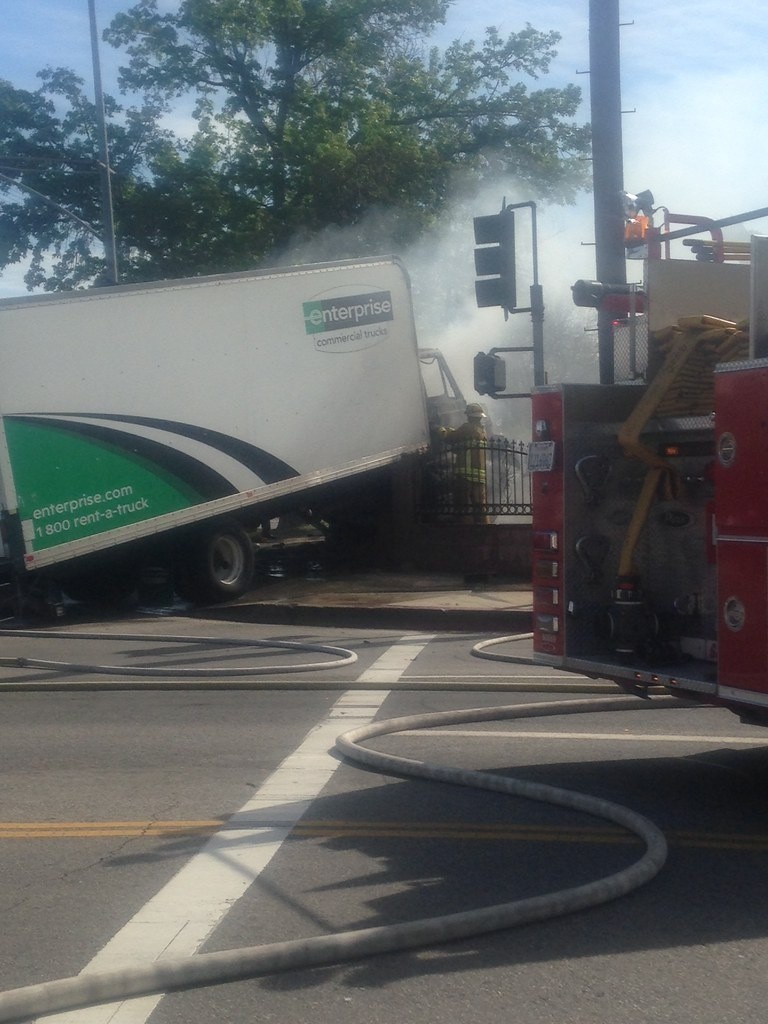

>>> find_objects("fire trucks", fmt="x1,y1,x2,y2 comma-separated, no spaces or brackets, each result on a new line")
519,194,768,722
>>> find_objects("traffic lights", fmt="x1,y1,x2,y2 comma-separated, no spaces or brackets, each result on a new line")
472,205,518,312
472,352,507,397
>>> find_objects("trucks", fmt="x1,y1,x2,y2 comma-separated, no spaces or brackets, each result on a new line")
0,258,437,616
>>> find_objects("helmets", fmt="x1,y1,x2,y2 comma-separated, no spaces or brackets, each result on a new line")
464,403,487,418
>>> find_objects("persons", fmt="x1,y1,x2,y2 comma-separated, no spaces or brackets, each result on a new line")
432,400,491,524
